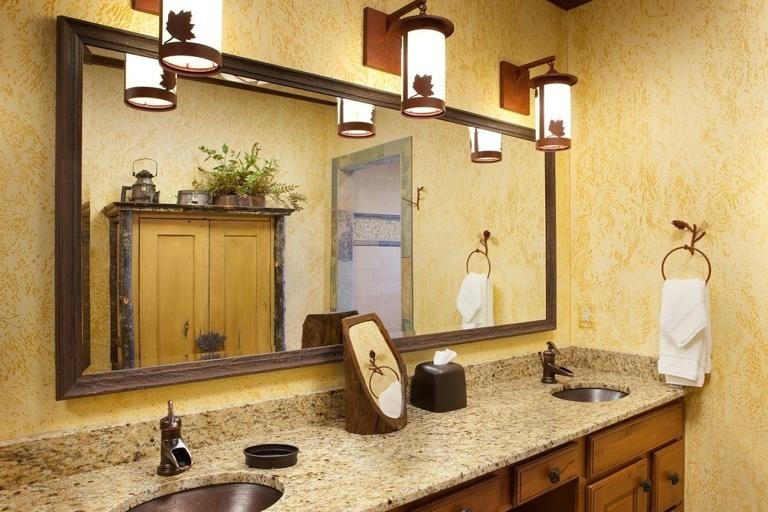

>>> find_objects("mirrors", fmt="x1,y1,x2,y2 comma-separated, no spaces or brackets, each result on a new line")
54,13,557,400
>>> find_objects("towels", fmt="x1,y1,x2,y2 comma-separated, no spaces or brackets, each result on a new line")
658,275,712,388
456,274,492,329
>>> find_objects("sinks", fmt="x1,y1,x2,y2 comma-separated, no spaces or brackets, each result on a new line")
549,379,633,404
115,471,287,512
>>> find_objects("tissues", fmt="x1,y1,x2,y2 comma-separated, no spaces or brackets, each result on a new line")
409,347,470,412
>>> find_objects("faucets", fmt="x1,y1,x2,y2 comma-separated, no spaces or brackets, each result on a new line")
156,397,196,476
536,338,576,384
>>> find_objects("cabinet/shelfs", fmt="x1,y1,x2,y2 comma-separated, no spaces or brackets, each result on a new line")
580,395,688,512
100,201,293,370
391,436,586,512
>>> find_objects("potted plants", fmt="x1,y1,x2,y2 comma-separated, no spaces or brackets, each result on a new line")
240,166,309,212
194,142,244,209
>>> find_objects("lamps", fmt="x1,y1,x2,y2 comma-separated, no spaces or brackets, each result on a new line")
468,124,502,165
131,0,223,77
336,97,374,138
499,56,578,154
363,0,454,120
125,50,178,109
